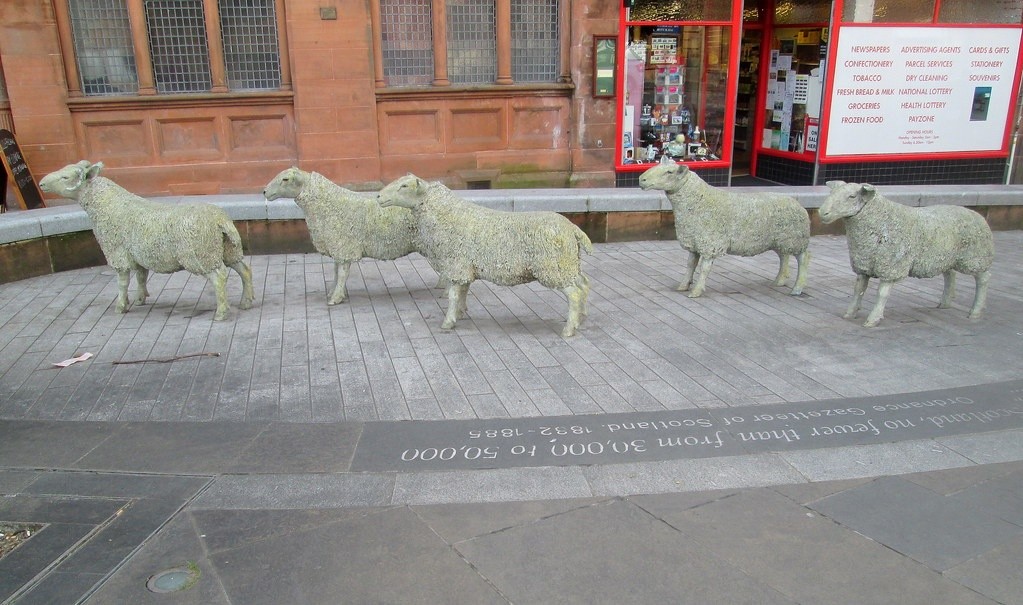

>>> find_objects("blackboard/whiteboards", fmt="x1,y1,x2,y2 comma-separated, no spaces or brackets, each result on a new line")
0,129,46,212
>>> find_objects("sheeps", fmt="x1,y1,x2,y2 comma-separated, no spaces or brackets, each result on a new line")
263,166,447,305
818,180,994,328
638,155,810,298
39,159,255,322
377,171,594,337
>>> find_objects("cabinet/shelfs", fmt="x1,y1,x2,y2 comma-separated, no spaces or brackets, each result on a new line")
703,69,753,154
